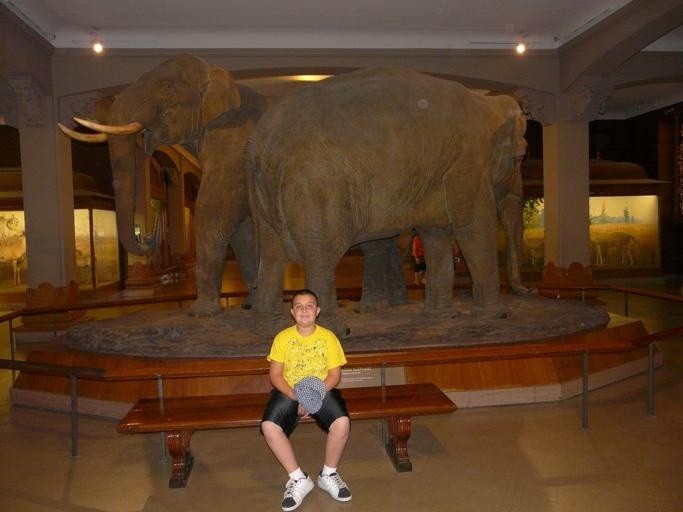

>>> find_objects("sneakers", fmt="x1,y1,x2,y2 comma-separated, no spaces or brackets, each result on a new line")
282,472,315,511
317,469,353,502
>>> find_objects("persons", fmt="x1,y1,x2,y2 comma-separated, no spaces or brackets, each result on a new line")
594,241,603,265
260,289,355,512
408,233,425,288
619,236,638,265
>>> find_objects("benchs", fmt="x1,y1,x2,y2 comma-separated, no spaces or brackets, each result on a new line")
116,382,458,488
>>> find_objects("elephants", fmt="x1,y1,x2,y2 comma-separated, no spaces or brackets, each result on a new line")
242,67,539,341
56,54,409,318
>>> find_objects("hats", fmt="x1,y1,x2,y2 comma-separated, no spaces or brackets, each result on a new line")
293,376,326,414
